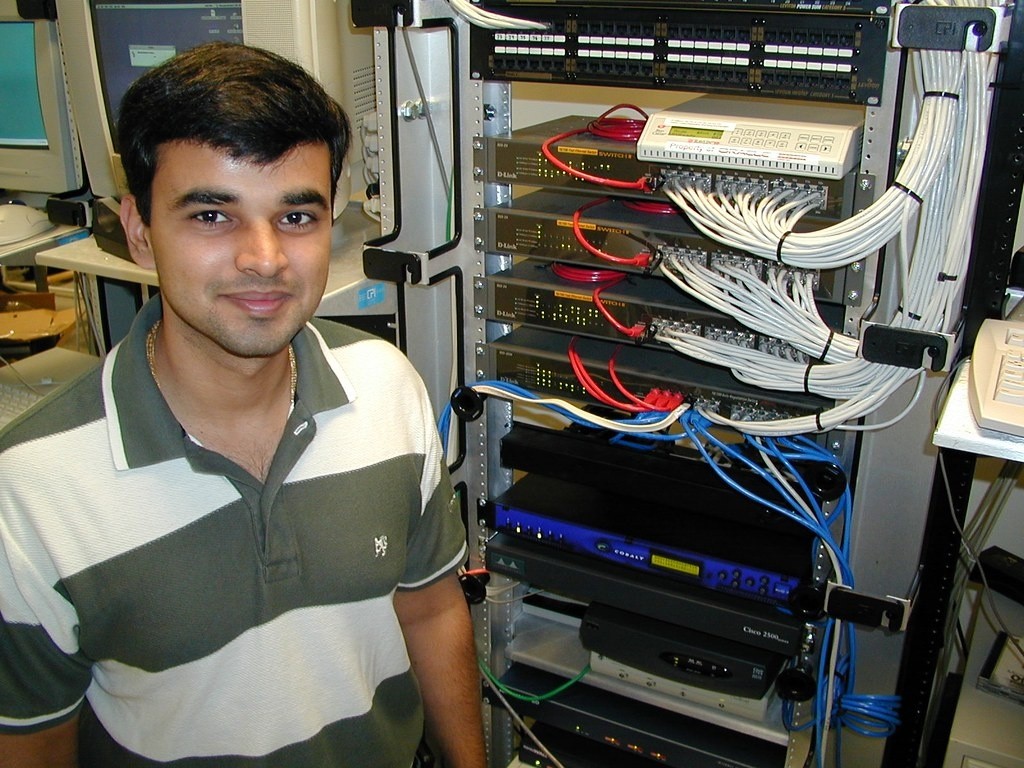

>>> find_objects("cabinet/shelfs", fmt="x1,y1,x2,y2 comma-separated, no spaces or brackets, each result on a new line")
941,587,1024,768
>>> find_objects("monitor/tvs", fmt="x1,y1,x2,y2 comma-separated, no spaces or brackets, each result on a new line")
55,0,379,219
0,0,84,207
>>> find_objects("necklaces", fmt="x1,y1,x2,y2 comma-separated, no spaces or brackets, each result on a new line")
146,320,297,400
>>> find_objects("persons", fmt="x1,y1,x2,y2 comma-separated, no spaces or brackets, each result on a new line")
0,41,488,768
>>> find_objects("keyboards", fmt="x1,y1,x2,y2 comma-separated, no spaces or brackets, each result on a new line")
971,317,1024,438
